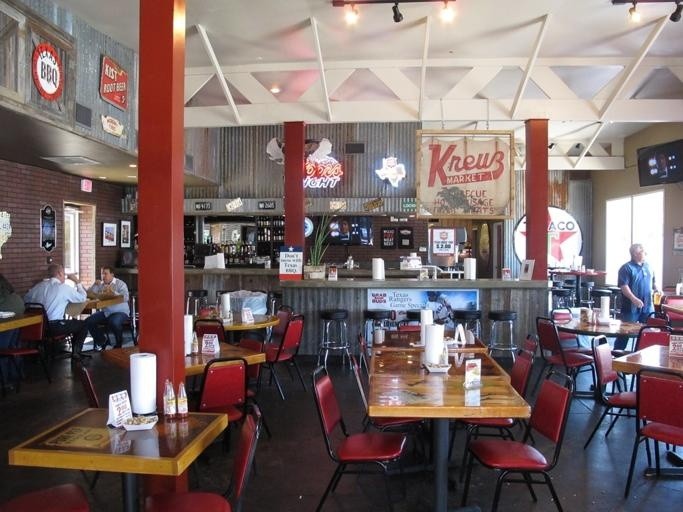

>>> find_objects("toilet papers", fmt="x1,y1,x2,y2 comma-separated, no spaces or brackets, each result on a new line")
130,353,157,414
420,309,434,346
184,315,193,354
464,258,476,279
220,292,230,318
426,324,445,363
204,253,226,270
600,296,610,320
372,258,385,280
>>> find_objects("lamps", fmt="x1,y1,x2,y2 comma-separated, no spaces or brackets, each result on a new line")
611,0,682,23
331,0,455,25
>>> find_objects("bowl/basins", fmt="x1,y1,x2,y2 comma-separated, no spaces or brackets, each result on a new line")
423,362,452,373
120,414,158,432
586,268,594,273
120,429,160,443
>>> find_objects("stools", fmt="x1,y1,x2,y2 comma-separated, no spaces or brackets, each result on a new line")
315,303,520,368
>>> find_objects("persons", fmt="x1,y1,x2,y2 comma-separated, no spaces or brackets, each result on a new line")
327,217,361,245
612,243,664,362
86,266,130,352
426,290,456,329
653,151,669,180
0,275,26,316
23,263,92,364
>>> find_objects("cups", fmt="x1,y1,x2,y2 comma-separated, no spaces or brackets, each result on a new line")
373,328,385,344
653,291,661,306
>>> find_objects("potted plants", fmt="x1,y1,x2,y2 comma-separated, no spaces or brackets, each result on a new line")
302,207,342,281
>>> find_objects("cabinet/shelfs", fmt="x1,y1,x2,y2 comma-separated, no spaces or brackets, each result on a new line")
255,214,284,265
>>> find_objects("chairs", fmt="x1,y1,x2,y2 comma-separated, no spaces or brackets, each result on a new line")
529,267,682,500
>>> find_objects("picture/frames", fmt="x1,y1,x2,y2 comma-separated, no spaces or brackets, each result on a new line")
99,220,118,248
118,218,132,250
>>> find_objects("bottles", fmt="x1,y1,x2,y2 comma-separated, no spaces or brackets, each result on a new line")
257,228,285,241
162,377,188,422
224,254,252,265
591,309,599,325
162,418,190,454
651,272,656,290
264,255,271,269
257,215,284,226
211,239,255,255
228,306,233,322
190,331,198,356
345,252,353,270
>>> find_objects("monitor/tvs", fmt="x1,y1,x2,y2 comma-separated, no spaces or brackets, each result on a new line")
637,139,683,187
318,216,374,246
